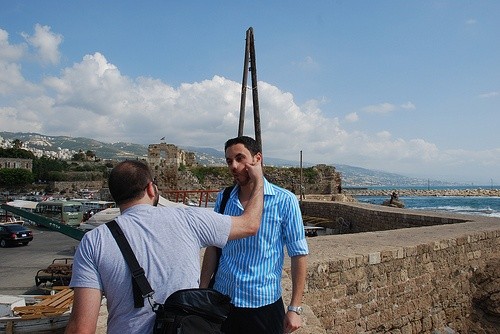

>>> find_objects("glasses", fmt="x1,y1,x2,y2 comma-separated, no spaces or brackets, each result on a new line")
143,179,157,191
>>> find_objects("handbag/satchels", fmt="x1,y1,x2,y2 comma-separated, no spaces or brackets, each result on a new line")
151,288,238,334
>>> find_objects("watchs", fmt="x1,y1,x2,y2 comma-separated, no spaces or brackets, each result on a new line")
288,306,303,315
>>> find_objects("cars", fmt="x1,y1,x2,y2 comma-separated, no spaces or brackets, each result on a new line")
0,223,33,248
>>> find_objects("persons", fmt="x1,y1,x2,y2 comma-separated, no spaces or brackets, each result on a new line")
63,157,264,334
199,136,308,334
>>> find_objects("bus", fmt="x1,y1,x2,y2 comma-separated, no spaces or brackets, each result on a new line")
17,188,117,228
